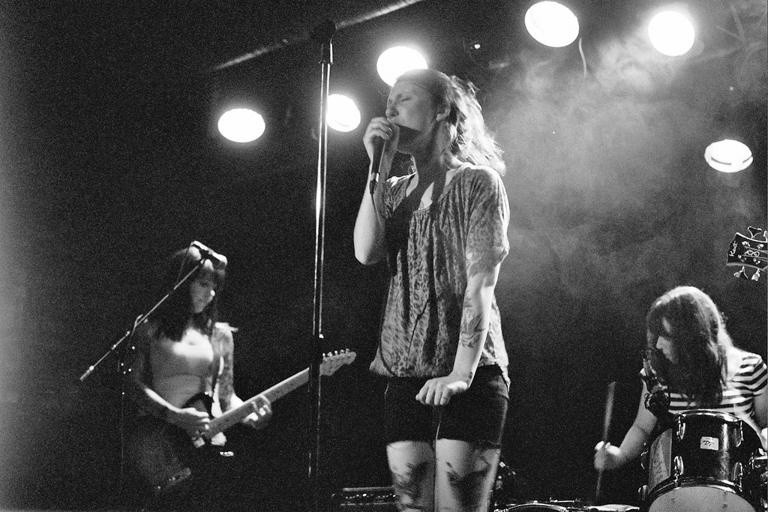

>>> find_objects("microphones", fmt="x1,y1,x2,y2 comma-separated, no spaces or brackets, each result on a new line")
192,240,228,271
639,354,663,394
370,123,387,195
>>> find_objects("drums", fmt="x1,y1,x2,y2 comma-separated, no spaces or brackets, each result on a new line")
494,497,599,511
333,485,394,506
638,409,768,512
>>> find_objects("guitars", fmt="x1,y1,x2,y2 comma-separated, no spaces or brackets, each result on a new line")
126,349,357,497
728,227,767,281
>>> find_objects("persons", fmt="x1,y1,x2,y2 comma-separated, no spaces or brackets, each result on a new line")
123,240,276,508
351,69,520,511
590,280,767,474
